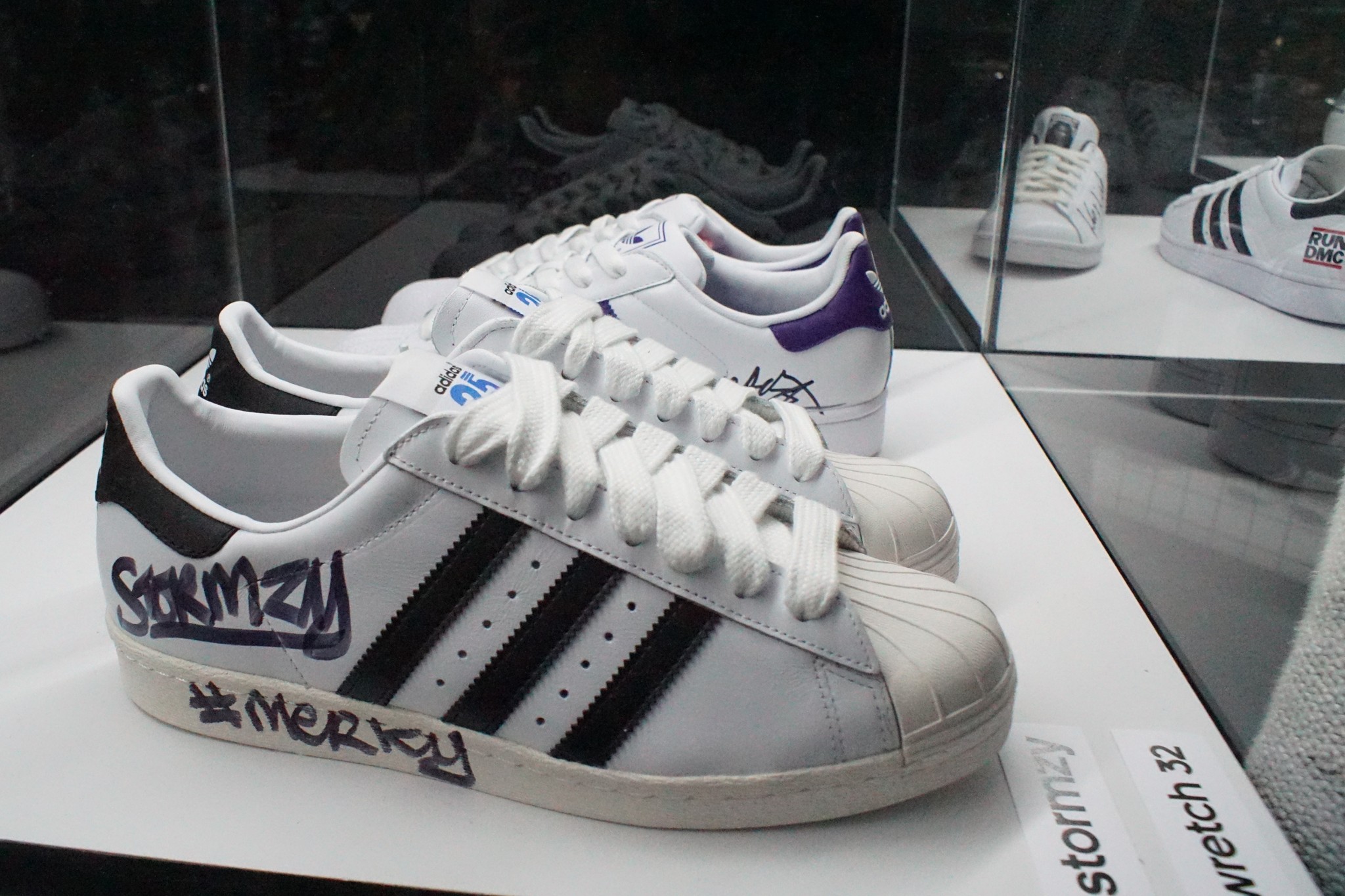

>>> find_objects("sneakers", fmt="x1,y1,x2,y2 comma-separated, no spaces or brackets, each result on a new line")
1207,360,1345,496
1146,355,1231,427
376,192,869,330
93,360,1019,833
1121,77,1228,192
423,95,838,284
1046,74,1137,187
320,221,897,461
190,266,962,592
1156,142,1345,326
971,105,1108,271
